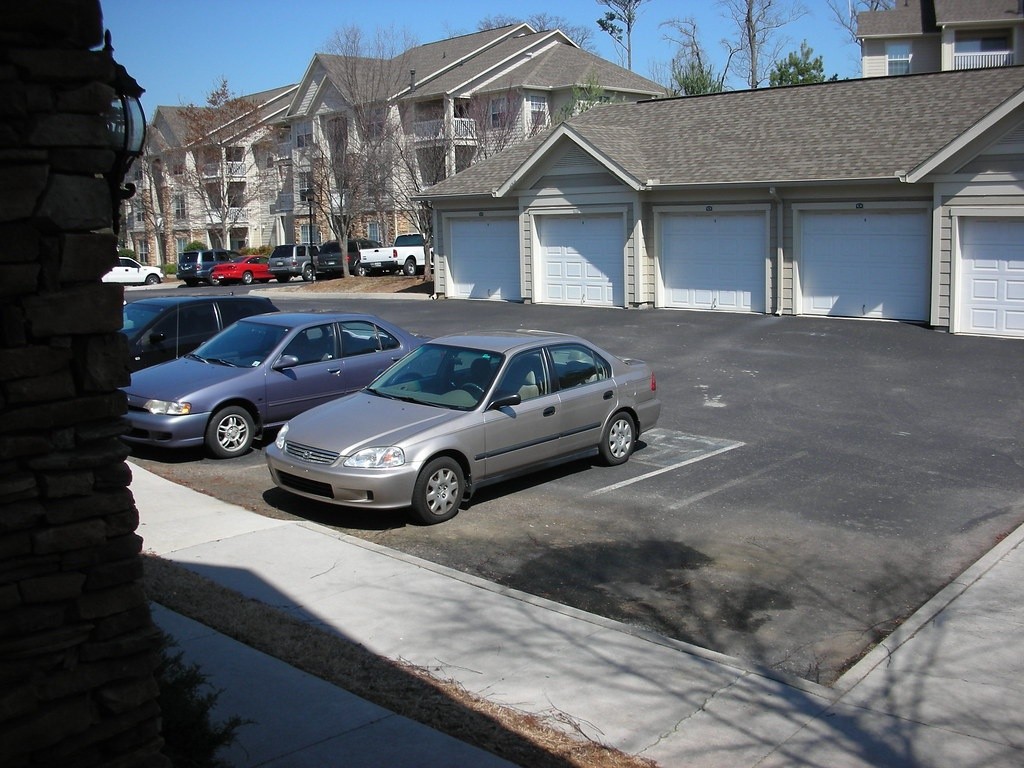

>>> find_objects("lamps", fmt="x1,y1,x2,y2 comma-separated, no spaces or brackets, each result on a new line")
102,30,148,234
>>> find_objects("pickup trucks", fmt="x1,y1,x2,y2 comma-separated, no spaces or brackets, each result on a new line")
358,233,435,276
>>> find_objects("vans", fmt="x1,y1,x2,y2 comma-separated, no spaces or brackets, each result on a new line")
177,249,242,284
118,295,281,372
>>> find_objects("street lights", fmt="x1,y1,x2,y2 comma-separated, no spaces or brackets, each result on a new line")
305,188,316,282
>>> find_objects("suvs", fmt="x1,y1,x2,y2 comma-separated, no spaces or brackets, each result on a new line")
267,244,325,282
101,256,164,286
317,239,383,277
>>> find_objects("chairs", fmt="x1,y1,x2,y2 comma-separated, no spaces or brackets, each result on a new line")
294,334,316,355
466,356,492,387
507,359,537,402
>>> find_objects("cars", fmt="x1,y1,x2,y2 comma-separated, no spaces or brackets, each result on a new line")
264,333,662,523
117,312,456,458
213,256,276,285
